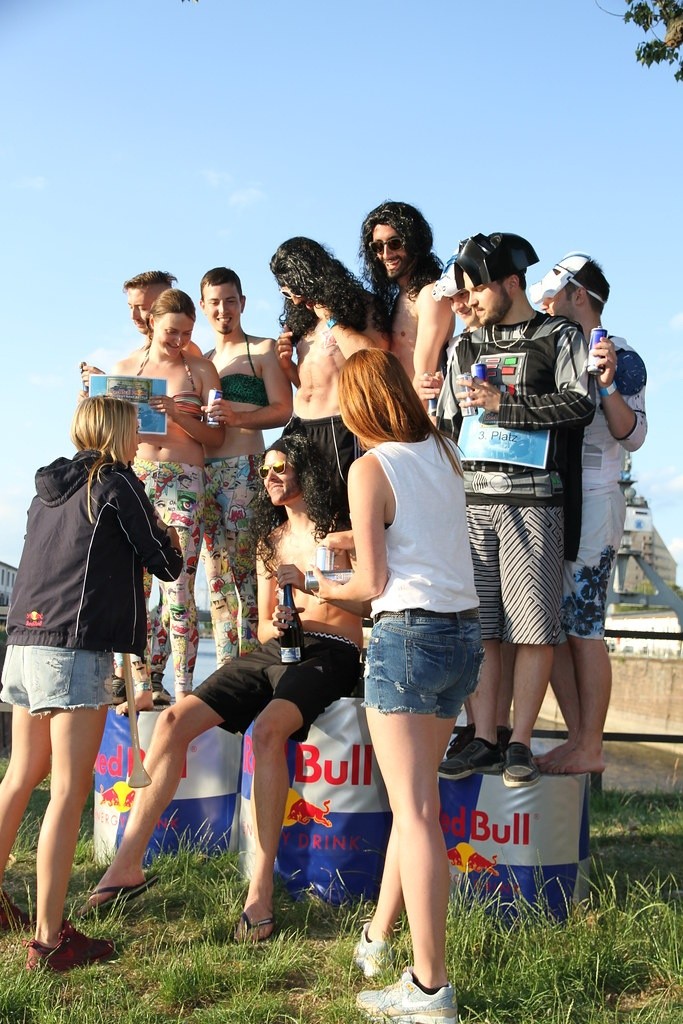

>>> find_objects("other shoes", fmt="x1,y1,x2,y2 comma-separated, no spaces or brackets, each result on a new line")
446,723,513,758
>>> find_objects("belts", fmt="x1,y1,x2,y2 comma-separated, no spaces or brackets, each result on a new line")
375,607,478,621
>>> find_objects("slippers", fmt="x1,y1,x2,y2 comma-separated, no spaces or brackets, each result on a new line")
235,912,275,946
77,874,158,918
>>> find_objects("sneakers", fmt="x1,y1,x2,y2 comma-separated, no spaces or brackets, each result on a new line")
357,968,458,1024
110,674,127,703
437,740,505,780
22,919,114,974
354,921,390,977
503,744,540,788
150,671,171,704
0,891,37,929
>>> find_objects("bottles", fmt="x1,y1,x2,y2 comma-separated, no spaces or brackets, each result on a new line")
279,584,304,665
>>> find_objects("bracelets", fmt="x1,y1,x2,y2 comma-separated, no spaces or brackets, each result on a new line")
327,319,338,329
164,526,176,533
600,381,617,396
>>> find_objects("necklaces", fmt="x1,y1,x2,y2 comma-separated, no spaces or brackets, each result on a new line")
492,308,534,348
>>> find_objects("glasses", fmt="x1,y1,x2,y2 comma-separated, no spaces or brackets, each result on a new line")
369,237,406,255
258,460,294,479
281,287,304,299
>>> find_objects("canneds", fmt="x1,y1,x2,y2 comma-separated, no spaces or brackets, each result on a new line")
456,361,489,416
305,545,354,591
207,388,223,425
587,326,608,375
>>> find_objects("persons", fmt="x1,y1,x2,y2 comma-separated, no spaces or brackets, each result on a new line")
199,266,294,712
525,253,647,774
71,435,368,947
106,289,224,716
355,199,457,428
81,271,206,707
415,232,600,791
0,395,185,973
270,235,393,528
308,348,488,1024
